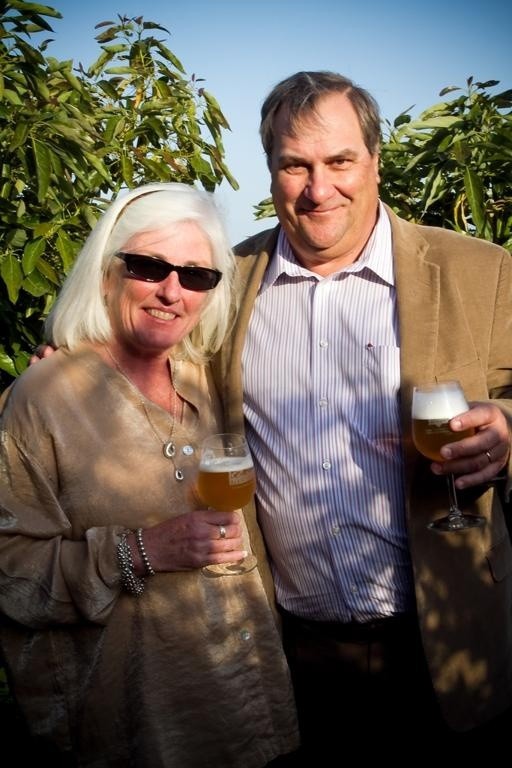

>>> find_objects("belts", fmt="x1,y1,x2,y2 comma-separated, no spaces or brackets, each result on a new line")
275,601,421,646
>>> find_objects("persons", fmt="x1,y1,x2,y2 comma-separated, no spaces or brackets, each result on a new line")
29,71,512,768
0,180,302,767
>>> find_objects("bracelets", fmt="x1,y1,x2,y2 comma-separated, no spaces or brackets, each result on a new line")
136,526,157,577
117,527,146,598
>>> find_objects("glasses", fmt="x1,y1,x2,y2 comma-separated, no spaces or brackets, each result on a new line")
115,250,221,293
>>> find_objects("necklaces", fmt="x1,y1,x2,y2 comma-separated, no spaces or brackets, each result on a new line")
104,344,187,480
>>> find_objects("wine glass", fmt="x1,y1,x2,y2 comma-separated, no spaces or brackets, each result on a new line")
409,379,488,534
195,431,258,576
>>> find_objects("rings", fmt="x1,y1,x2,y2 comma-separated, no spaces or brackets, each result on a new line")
484,450,494,464
219,524,227,539
